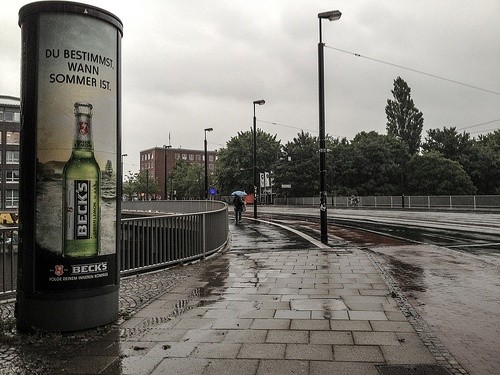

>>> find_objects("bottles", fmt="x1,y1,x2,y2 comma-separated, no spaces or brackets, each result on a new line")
61,102,100,259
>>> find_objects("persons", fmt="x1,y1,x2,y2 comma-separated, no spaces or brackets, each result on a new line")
232,195,243,224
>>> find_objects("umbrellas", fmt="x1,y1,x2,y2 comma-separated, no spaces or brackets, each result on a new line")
231,191,248,196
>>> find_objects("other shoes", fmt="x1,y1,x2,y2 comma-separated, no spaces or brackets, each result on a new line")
239,220,241,222
236,221,238,225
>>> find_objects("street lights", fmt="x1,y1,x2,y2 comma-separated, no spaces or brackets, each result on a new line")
164,145,172,200
252,100,266,220
317,9,343,245
204,127,215,200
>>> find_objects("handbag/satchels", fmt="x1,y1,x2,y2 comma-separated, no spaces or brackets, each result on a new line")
242,205,247,211
